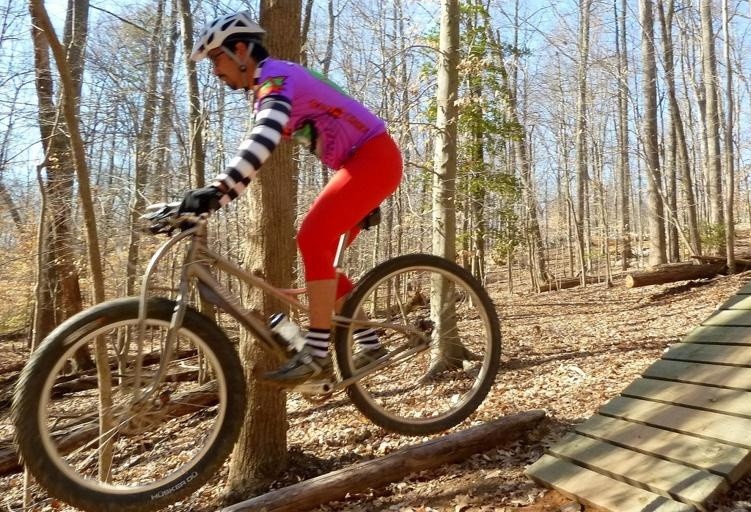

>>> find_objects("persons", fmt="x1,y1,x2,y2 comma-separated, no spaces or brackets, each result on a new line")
134,8,407,386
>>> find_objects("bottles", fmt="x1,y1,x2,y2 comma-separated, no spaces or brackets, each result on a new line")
267,312,308,351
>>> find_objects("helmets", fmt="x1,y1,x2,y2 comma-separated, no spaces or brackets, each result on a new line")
189,10,267,62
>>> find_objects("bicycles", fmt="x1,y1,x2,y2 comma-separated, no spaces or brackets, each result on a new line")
11,204,502,511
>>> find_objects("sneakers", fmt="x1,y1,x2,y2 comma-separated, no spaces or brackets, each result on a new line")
352,345,388,371
262,347,335,386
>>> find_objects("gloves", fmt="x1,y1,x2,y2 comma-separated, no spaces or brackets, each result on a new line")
177,185,225,232
